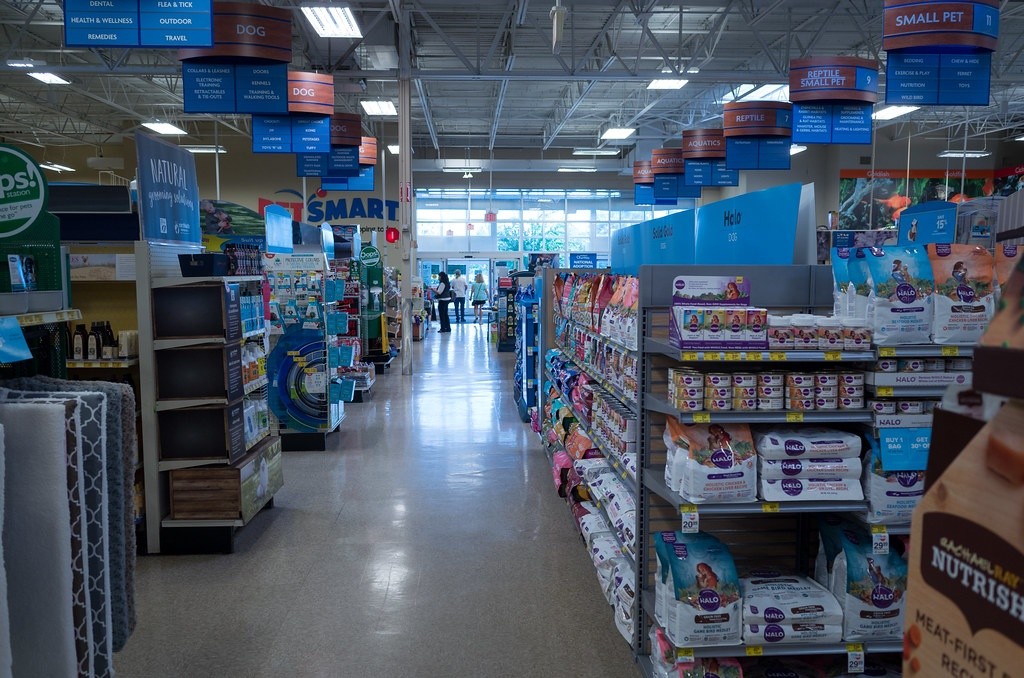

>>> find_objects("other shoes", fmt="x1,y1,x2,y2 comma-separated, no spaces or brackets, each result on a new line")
438,328,451,332
479,321,483,325
461,319,466,322
456,320,459,323
473,318,478,323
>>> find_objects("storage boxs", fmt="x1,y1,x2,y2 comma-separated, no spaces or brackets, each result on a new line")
324,277,346,300
47,184,139,243
327,310,348,335
155,252,284,520
329,344,354,369
330,375,356,403
673,305,770,341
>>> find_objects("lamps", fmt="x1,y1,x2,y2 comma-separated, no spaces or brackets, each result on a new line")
791,144,808,156
179,145,228,153
141,117,188,135
360,96,398,116
936,150,993,159
7,58,72,85
388,143,399,155
442,167,482,172
557,166,597,173
572,148,620,156
39,161,76,172
601,127,636,140
300,0,364,39
872,104,921,120
647,66,700,90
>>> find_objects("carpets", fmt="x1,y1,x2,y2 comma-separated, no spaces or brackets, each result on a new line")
0,379,138,678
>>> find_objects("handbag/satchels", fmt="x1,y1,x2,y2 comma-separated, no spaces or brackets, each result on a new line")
449,290,456,302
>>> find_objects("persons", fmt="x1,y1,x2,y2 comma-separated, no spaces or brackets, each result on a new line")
469,274,489,325
452,269,468,322
431,272,452,333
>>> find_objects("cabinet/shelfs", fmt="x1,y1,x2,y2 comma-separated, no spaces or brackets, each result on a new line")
334,240,429,402
488,277,541,439
267,263,346,452
542,267,639,667
634,262,996,677
47,184,284,555
0,248,83,380
